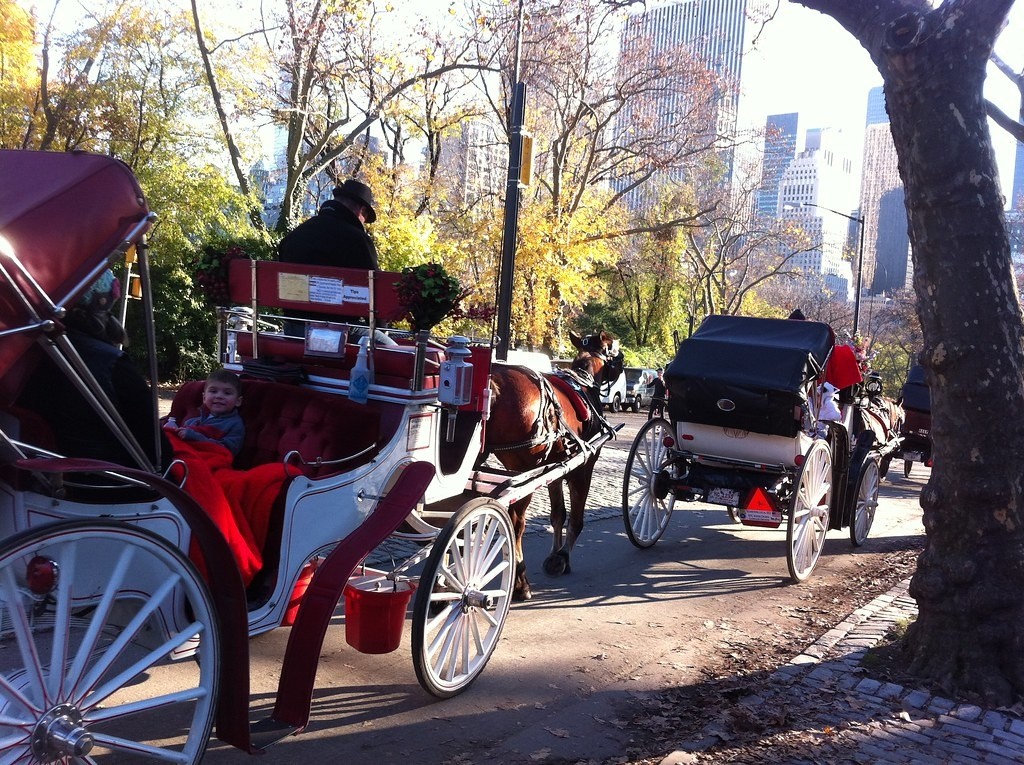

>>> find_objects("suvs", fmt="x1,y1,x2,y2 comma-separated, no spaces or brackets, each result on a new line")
621,367,670,413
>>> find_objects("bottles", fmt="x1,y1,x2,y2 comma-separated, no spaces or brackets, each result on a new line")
347,346,371,404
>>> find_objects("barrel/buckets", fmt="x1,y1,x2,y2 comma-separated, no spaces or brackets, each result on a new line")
343,543,416,654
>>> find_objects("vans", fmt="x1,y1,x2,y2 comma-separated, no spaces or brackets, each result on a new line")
550,359,626,413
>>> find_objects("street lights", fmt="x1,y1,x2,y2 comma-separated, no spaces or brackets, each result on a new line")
783,201,866,337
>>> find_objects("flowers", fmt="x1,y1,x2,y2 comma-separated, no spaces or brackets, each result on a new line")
180,231,270,308
844,334,880,376
390,261,496,334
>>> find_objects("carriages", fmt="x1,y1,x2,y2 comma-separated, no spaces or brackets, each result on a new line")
622,314,907,583
0,148,627,765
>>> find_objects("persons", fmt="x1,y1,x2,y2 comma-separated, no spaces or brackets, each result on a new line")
646,368,667,421
66,269,127,345
279,180,397,345
176,369,245,456
907,350,926,382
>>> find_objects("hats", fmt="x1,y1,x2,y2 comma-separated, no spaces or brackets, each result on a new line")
332,179,376,223
655,368,663,371
71,268,120,311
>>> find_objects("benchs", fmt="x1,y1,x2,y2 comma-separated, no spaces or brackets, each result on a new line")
0,399,188,504
162,380,379,495
223,247,447,395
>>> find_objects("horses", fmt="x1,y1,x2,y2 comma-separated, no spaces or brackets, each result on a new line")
475,330,623,600
856,398,906,468
